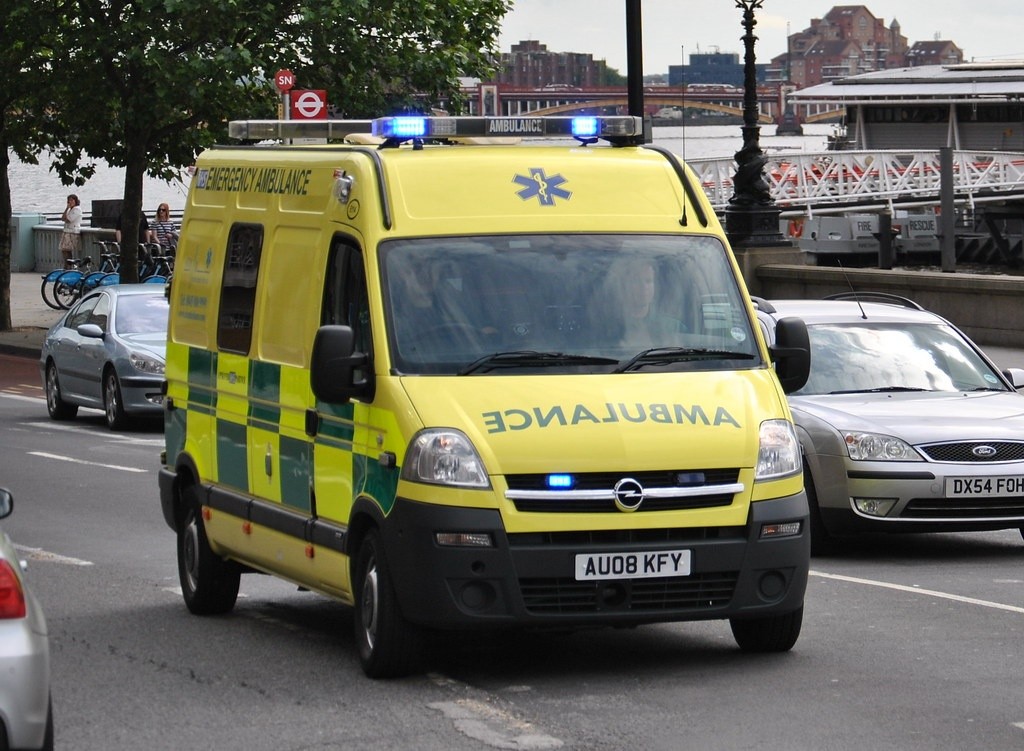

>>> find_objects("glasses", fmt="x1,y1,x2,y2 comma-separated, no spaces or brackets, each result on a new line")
158,208,166,212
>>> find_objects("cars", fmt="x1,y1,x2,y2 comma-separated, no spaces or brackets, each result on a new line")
703,291,1024,556
0,486,55,751
39,283,170,430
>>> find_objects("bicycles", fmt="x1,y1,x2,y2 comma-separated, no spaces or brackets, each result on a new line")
41,241,175,310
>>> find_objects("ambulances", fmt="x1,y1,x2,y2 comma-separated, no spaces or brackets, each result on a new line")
157,112,812,684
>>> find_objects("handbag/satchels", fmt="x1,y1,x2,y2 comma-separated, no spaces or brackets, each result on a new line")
170,243,177,257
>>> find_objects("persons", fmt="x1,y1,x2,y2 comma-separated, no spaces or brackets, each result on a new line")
392,248,505,359
152,203,178,257
586,257,689,356
60,195,82,271
117,208,150,276
